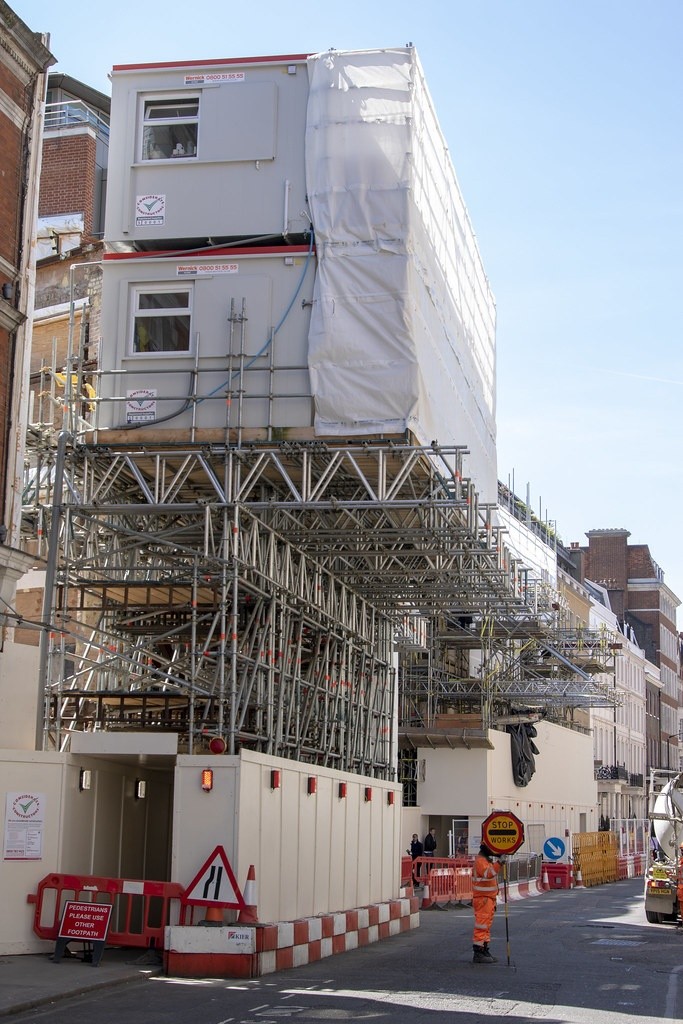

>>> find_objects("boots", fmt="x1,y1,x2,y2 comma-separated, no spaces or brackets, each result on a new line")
473,942,497,963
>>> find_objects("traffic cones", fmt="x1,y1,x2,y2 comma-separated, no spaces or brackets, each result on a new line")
572,866,586,889
419,876,432,910
540,867,551,892
227,864,267,926
196,906,226,927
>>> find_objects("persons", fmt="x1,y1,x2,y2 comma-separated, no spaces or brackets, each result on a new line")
457,828,468,859
406,833,424,888
472,840,508,962
423,828,437,875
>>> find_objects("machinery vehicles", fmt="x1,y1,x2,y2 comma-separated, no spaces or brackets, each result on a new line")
641,767,683,924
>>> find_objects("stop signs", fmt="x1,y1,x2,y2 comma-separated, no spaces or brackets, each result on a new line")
481,810,526,854
